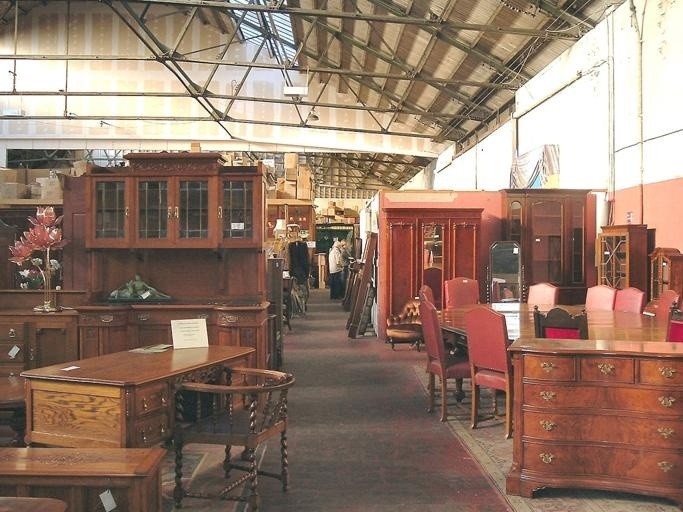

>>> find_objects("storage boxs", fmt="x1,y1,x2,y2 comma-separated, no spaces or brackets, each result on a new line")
315,201,358,225
266,153,315,200
190,144,262,166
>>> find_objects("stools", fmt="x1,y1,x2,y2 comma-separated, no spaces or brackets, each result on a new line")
0,495,70,512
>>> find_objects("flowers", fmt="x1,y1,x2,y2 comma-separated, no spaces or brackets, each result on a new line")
7,205,70,313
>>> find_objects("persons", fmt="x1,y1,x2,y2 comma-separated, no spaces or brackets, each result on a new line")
339,238,350,291
286,228,310,310
328,240,348,299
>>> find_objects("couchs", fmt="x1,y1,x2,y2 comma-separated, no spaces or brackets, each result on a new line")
385,297,429,351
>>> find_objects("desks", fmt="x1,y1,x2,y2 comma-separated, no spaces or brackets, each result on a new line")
18,345,257,464
0,447,167,512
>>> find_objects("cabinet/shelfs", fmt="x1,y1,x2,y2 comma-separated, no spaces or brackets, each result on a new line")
648,247,683,309
287,205,312,240
504,337,683,505
84,176,134,249
267,202,285,238
503,188,593,302
78,300,270,369
0,307,79,371
601,223,650,289
133,174,216,249
380,208,486,343
217,177,261,248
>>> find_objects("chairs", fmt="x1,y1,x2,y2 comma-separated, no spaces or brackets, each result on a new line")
613,287,648,314
665,303,683,343
172,368,294,509
585,284,617,311
658,289,680,313
464,307,514,441
419,301,499,426
443,277,480,356
528,282,560,305
533,305,589,339
418,284,483,385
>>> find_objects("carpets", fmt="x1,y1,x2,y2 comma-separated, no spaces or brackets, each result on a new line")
157,439,267,512
407,362,681,512
